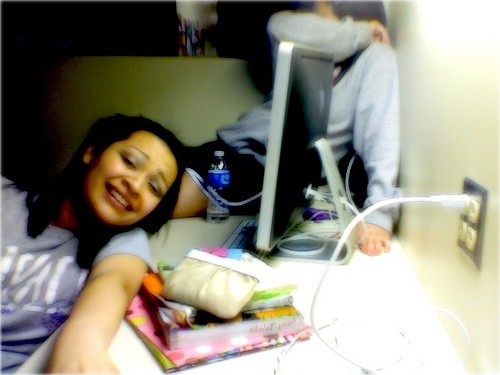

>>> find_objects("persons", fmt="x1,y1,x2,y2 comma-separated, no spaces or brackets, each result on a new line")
0,114,189,375
168,0,400,255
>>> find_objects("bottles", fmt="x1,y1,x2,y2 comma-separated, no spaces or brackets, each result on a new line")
206,150,230,224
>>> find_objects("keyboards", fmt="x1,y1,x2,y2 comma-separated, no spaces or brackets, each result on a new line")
217,217,267,261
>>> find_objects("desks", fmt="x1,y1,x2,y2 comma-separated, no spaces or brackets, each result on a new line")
10,214,467,374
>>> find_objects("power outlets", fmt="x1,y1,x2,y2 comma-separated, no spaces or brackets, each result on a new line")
456,176,490,269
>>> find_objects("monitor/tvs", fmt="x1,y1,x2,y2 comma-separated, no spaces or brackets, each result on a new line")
254,41,336,255
